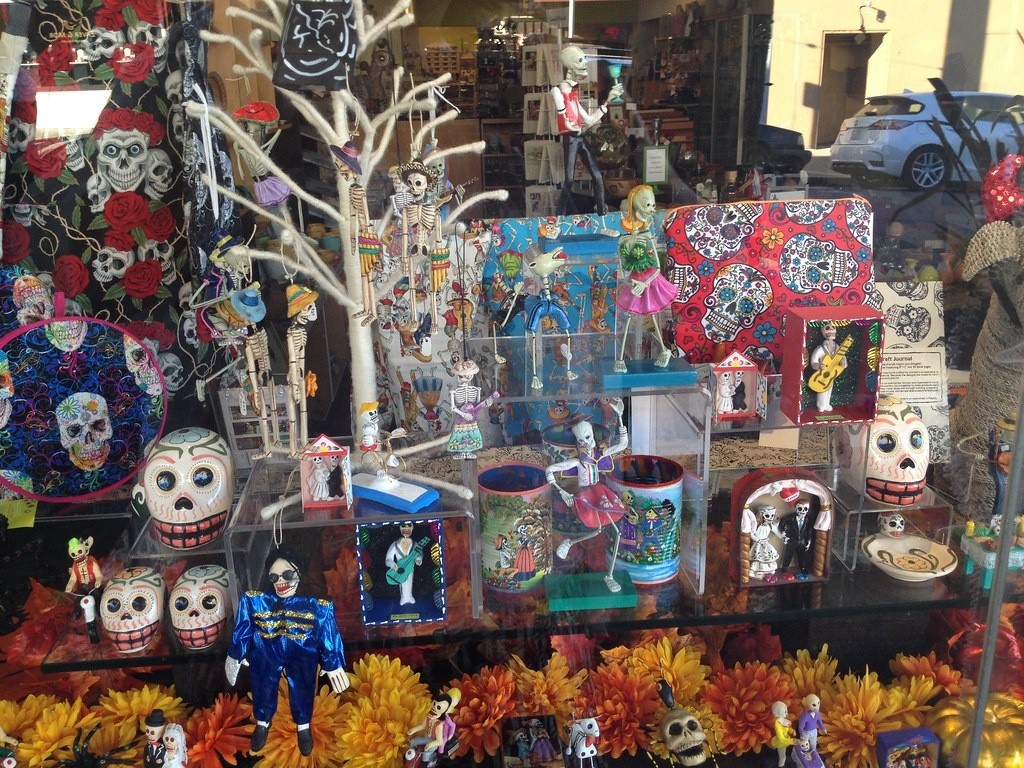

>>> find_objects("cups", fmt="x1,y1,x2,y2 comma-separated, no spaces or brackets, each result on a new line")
478,462,553,597
540,421,614,538
603,455,683,585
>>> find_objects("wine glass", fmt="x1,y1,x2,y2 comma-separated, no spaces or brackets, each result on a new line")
607,63,624,104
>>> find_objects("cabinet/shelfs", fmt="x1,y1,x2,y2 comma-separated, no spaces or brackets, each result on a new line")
653,8,775,169
317,45,525,228
40,329,1024,768
216,375,299,470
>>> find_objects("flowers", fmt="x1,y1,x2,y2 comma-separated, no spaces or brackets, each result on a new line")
1,509,1024,767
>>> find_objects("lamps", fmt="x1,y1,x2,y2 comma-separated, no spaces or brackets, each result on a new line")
859,5,887,33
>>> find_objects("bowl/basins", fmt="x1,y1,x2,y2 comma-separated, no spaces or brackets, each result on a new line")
861,532,958,582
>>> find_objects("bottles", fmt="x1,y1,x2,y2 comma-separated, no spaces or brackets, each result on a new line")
937,253,955,284
719,170,740,203
875,221,905,271
905,258,919,285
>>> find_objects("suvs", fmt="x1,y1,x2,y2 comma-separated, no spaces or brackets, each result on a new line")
828,90,1023,191
